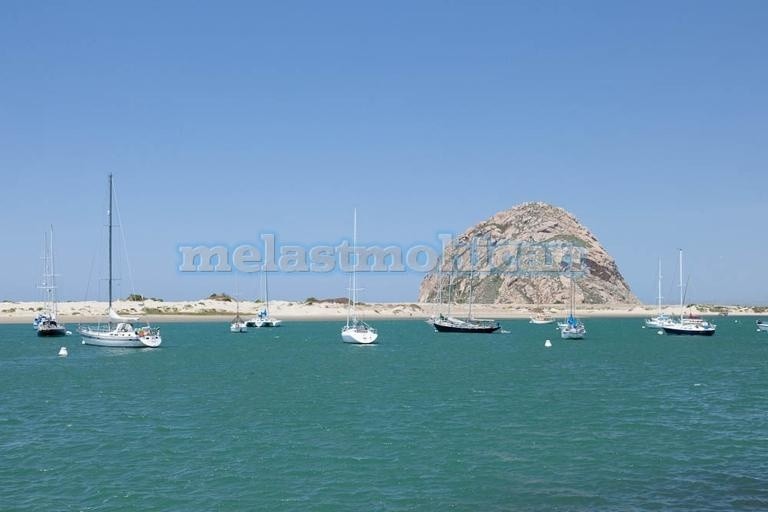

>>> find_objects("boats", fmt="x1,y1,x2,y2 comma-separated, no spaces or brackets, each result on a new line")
756,319,768,332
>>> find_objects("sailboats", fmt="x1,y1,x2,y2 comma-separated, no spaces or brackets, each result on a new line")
528,283,554,324
557,268,586,339
246,260,281,327
644,246,717,336
32,224,67,336
341,208,378,346
431,235,501,334
231,272,248,333
78,171,164,348
